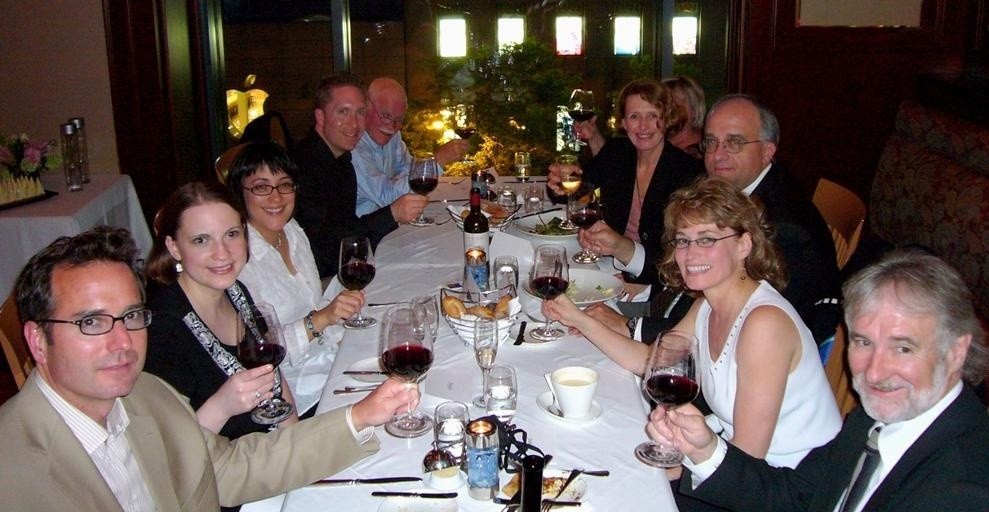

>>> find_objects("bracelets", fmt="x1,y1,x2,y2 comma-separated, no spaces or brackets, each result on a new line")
305,310,323,344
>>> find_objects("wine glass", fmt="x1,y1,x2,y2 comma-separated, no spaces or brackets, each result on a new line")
379,303,435,439
566,88,595,145
432,399,469,462
524,184,544,214
633,330,701,470
557,155,582,229
336,236,378,329
515,152,532,193
529,244,570,342
470,317,498,409
485,365,519,423
493,255,519,296
238,302,297,425
408,152,439,226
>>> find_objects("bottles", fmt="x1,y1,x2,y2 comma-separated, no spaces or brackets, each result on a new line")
464,248,490,300
60,117,91,192
464,187,491,278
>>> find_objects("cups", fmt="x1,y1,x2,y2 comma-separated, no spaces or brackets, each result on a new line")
465,420,501,502
551,366,599,419
424,468,463,490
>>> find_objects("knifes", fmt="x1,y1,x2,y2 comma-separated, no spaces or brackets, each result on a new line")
370,490,458,502
307,476,424,485
513,320,528,346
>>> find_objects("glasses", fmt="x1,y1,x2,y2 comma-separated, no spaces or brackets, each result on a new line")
35,307,153,336
667,234,739,250
366,95,410,126
242,183,301,197
699,134,764,154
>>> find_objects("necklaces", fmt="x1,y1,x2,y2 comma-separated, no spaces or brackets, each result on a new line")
269,234,284,249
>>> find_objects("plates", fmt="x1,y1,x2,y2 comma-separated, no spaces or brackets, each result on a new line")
345,356,432,385
519,268,626,306
515,210,579,240
568,182,601,265
535,391,603,430
496,467,587,512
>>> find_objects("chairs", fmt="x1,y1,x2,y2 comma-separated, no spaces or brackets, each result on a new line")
214,142,253,187
154,206,165,239
825,325,859,418
812,178,866,271
245,111,289,152
0,289,34,392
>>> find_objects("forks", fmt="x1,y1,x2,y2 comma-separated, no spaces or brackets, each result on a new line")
540,498,582,510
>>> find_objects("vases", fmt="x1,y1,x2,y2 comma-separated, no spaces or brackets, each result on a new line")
4,163,40,182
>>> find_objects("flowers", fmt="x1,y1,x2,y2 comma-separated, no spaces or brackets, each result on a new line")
0,133,58,173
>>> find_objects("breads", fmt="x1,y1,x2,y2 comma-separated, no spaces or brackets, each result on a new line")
443,295,514,318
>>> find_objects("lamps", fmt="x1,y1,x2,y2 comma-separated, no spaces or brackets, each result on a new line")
225,74,270,139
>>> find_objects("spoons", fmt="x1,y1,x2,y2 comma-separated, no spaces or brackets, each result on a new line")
544,372,562,418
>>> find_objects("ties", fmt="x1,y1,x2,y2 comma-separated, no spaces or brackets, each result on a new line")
837,426,882,512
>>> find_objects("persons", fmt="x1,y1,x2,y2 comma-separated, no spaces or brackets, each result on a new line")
548,80,710,249
285,75,430,279
575,76,707,163
541,179,843,469
647,256,989,511
0,226,421,512
144,185,298,440
568,93,845,345
350,78,472,219
224,139,366,417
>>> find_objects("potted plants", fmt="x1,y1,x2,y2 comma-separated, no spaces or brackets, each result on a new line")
415,38,601,176
